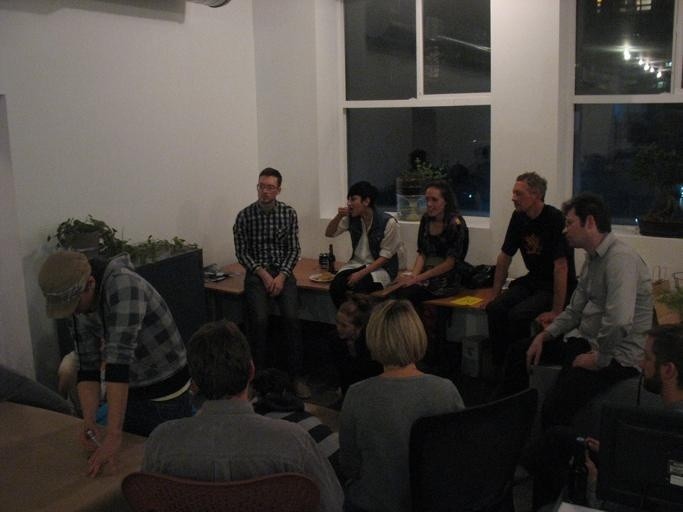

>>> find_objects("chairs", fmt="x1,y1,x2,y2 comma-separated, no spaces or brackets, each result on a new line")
408,388,538,511
120,472,320,512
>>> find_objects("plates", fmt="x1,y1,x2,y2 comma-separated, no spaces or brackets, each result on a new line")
307,273,337,283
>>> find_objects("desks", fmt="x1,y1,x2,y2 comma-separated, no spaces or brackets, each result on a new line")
0,401,148,512
204,258,505,377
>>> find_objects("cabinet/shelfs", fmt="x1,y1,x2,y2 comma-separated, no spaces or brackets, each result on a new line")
27,248,205,400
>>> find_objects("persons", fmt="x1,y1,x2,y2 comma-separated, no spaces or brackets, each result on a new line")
393,180,469,308
141,318,345,512
233,168,312,398
253,367,340,466
479,172,577,385
38,250,192,479
524,194,653,469
324,181,400,310
339,298,467,511
331,294,380,396
55,339,107,411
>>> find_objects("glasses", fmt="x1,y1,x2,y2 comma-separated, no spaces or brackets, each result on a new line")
258,183,278,193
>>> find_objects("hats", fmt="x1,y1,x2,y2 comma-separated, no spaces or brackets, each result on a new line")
37,251,92,320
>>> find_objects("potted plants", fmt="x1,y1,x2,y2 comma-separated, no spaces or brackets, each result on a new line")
622,142,683,238
